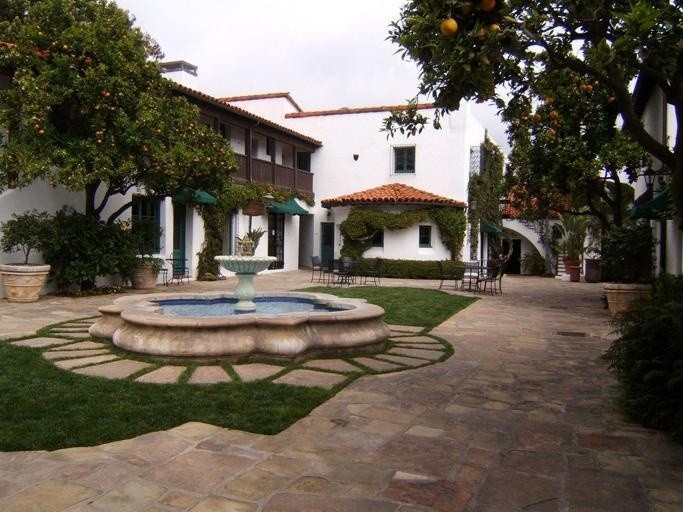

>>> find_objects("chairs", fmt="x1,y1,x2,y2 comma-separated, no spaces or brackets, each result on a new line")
308,254,384,289
156,267,169,288
436,257,506,298
169,249,189,286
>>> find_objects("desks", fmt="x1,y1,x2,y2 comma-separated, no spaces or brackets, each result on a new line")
165,258,188,285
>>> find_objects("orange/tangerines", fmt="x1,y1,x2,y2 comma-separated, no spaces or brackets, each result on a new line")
424,0,615,145
5,32,232,174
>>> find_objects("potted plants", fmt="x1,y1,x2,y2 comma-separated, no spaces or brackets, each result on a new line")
547,205,593,274
598,212,662,320
127,214,161,290
0,206,55,302
